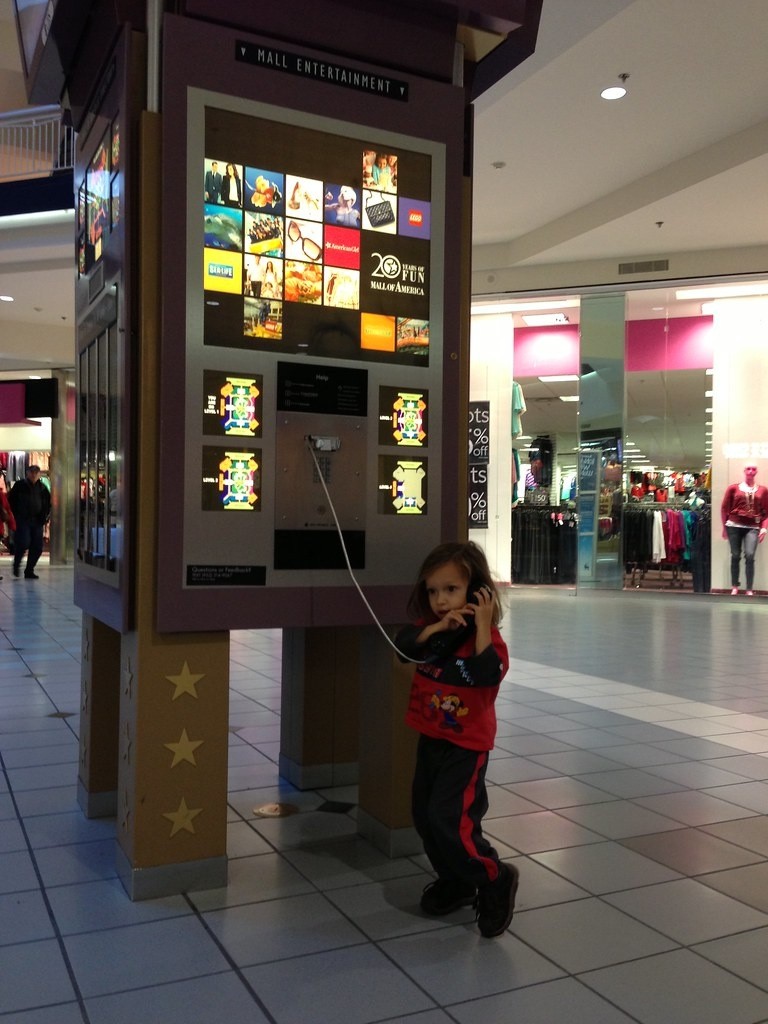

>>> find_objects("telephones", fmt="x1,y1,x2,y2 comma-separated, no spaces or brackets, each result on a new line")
425,582,489,660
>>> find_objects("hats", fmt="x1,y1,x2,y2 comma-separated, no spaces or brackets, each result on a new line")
27,466,40,472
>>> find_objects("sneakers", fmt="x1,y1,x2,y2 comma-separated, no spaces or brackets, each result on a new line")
24,571,39,579
13,563,20,577
422,875,479,915
475,863,519,938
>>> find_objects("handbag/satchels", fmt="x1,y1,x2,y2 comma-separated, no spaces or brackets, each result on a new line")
366,202,395,227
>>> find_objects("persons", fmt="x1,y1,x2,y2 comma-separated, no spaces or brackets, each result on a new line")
325,184,360,226
204,161,241,206
525,460,543,490
362,150,398,194
246,256,282,298
394,542,519,937
0,466,52,580
721,465,768,595
246,215,282,243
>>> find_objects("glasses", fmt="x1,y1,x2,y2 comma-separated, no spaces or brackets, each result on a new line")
289,221,322,260
